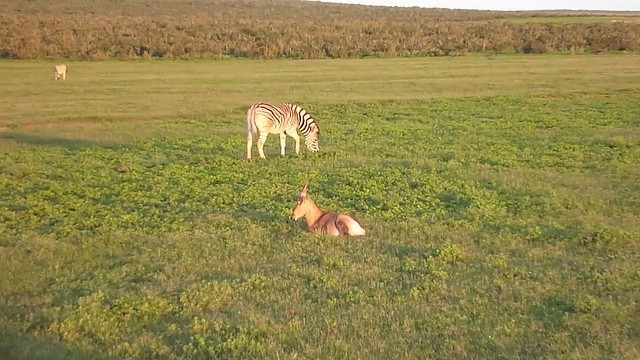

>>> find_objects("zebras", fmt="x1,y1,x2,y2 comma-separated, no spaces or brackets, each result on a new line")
246,102,320,160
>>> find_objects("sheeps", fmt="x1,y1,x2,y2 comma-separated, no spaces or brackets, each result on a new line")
288,182,366,237
